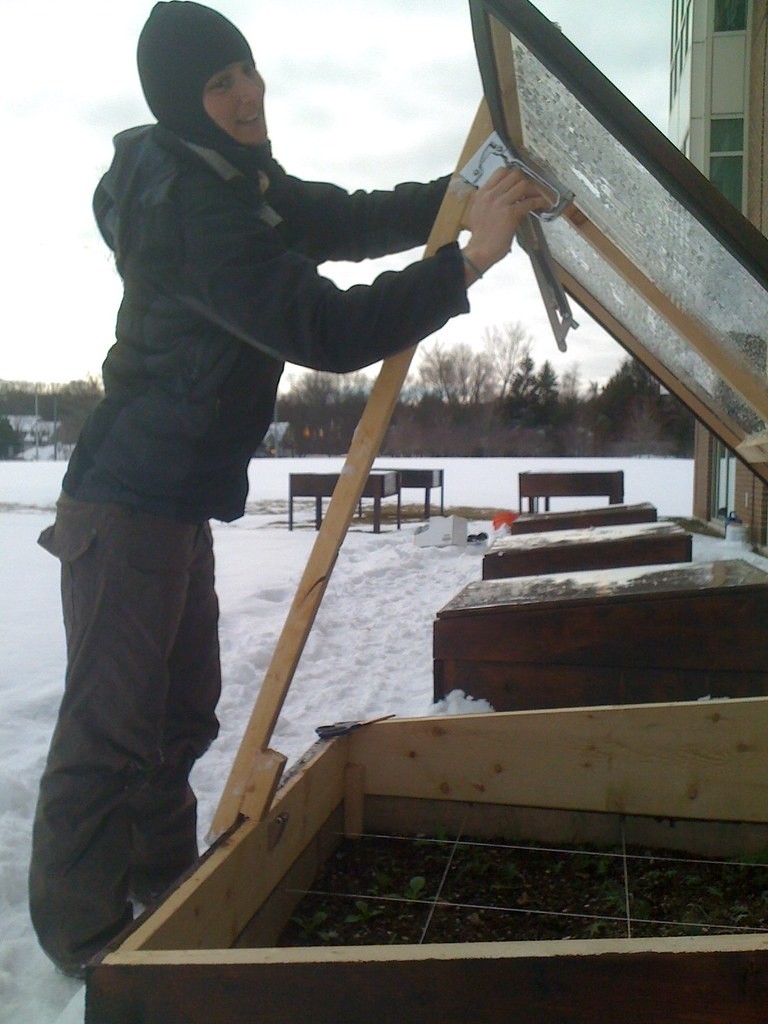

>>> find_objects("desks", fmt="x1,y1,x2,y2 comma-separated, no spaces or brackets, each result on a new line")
519,471,624,515
289,467,445,533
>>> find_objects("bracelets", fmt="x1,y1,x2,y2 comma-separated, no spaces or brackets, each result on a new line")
463,255,482,278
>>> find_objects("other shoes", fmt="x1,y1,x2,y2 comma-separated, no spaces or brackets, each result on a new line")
58,900,134,979
131,886,159,908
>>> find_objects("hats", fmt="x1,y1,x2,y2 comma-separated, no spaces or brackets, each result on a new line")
137,0,273,163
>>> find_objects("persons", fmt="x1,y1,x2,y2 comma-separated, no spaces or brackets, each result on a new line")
28,0,552,976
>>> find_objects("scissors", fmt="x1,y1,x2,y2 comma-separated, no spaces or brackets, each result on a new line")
316,712,396,741
